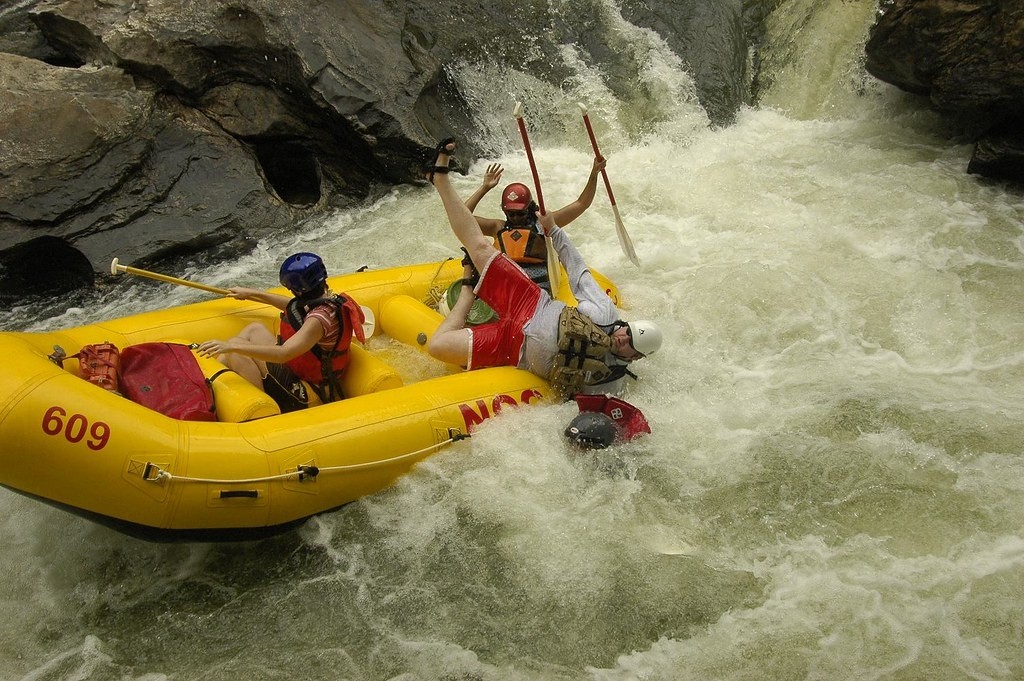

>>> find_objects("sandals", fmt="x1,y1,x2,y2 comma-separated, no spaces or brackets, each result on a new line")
461,247,480,299
429,137,455,185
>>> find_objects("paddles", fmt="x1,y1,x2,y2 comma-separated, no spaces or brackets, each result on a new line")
110,256,375,340
514,101,562,299
575,100,639,268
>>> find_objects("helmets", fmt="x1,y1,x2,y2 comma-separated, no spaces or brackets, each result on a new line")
564,412,615,449
279,252,328,288
502,183,532,210
628,320,663,355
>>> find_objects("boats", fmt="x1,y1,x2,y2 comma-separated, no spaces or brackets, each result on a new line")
0,257,621,543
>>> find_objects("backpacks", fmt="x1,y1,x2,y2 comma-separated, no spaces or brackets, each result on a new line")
120,342,216,423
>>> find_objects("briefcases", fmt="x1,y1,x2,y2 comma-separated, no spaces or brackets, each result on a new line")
80,341,120,395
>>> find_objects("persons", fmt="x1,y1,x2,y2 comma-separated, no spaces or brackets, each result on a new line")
422,137,662,390
464,155,606,294
196,252,366,413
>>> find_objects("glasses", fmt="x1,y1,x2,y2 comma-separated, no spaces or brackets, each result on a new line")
627,327,635,349
507,208,530,217
